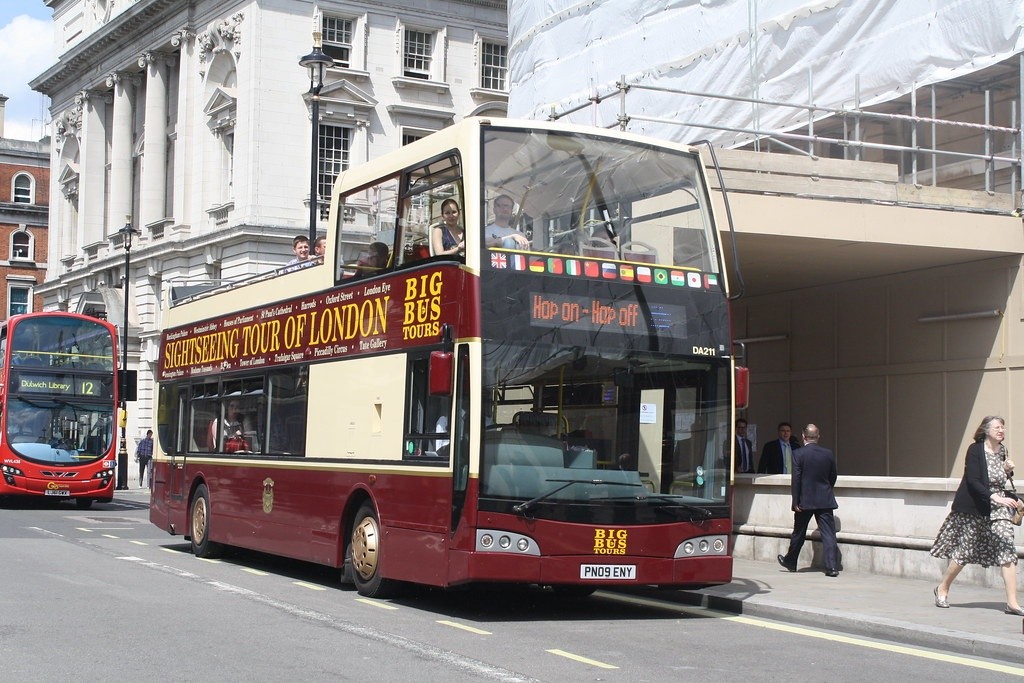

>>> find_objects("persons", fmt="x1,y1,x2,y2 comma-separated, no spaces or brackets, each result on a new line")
777,423,839,576
310,236,326,266
432,199,464,258
135,430,153,487
285,235,318,273
929,415,1024,616
757,422,801,474
211,399,253,453
723,418,754,474
435,414,450,457
354,242,389,276
485,194,530,252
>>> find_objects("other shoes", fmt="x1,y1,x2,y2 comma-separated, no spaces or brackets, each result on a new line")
1005,605,1024,615
934,585,949,607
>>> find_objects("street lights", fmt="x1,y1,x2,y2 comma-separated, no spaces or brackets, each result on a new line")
299,31,336,256
114,215,137,491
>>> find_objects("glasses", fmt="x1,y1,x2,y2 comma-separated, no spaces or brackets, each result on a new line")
992,426,1006,430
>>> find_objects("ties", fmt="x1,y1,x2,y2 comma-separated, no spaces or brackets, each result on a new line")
741,438,747,471
784,443,792,473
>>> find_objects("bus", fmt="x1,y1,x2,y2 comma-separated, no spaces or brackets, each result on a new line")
149,116,750,597
0,312,127,509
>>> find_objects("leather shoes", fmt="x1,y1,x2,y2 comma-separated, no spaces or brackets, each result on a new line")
826,568,839,575
778,555,797,571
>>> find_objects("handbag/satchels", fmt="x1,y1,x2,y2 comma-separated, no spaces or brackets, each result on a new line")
1005,448,1024,526
134,448,140,463
225,434,250,453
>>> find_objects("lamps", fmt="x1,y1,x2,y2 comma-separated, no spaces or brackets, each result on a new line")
733,334,789,344
105,76,116,88
138,56,148,68
917,310,1000,322
75,95,83,106
169,34,180,47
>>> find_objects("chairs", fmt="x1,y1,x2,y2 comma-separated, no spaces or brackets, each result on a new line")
339,238,660,277
7,350,105,370
168,410,305,458
173,257,325,305
